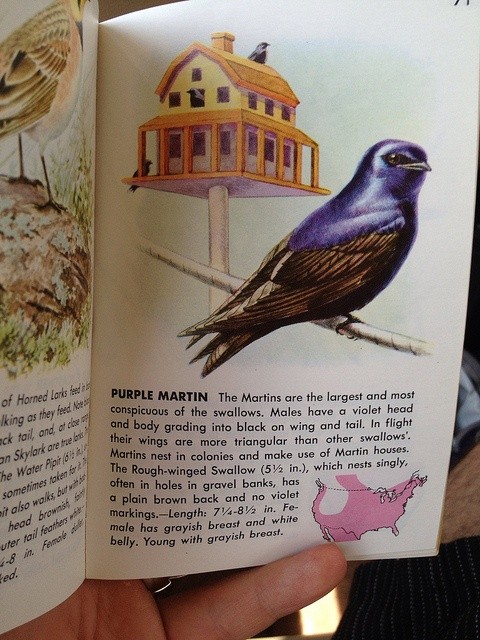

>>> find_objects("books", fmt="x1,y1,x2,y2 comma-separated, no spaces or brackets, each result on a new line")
0,1,478,639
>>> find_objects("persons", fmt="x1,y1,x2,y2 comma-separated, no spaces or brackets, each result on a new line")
0,542,346,640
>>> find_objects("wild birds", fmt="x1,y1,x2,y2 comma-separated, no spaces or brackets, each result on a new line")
187,87,205,107
247,42,271,64
177,138,432,379
0,0,88,216
129,159,153,193
